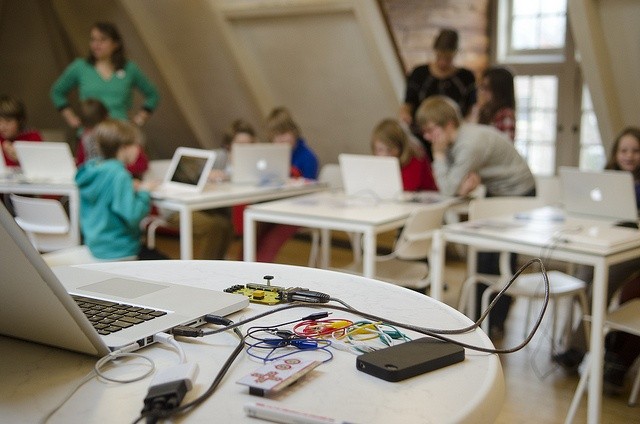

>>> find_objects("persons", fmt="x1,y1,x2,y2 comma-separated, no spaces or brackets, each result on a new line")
416,95,536,340
231,106,320,263
549,127,640,390
0,94,65,217
370,117,439,192
75,100,149,178
400,27,477,162
74,119,171,261
158,118,259,260
49,21,161,138
466,66,515,144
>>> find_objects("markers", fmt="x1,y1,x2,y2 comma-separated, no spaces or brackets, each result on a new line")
243,401,354,424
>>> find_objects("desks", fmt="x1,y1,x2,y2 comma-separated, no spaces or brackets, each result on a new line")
0,166,87,246
240,191,440,299
1,259,507,423
428,203,639,422
130,164,331,260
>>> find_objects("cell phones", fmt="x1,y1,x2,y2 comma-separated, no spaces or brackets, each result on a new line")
357,336,466,382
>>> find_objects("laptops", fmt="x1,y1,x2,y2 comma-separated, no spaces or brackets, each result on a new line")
337,152,404,201
2,198,251,360
16,140,76,184
229,143,288,187
559,166,634,224
147,147,216,199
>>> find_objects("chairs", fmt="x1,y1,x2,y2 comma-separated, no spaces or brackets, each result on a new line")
566,300,639,421
470,197,592,360
0,194,78,253
330,206,441,293
299,162,366,265
